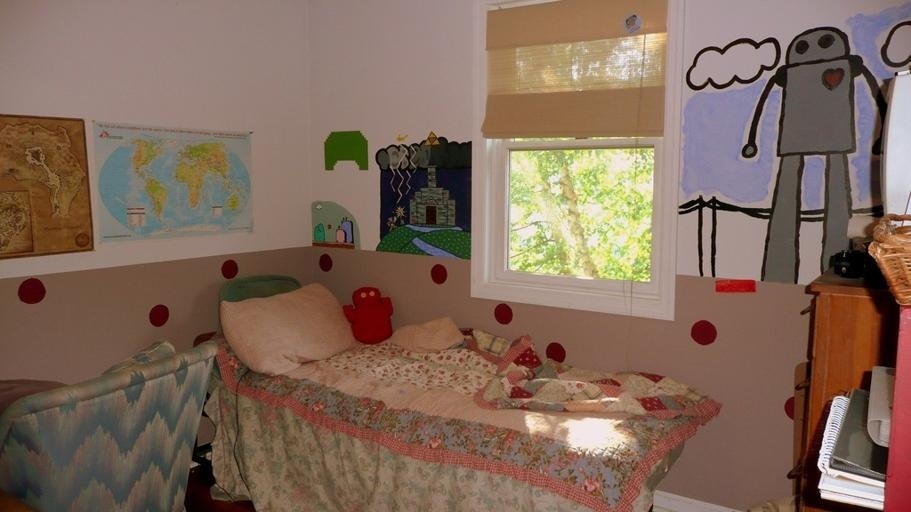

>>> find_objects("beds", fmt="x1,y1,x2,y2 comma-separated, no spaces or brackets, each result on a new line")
192,326,724,512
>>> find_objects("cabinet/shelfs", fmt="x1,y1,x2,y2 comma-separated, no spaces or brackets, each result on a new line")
786,267,894,511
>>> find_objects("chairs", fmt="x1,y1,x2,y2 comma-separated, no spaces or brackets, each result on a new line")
0,340,220,512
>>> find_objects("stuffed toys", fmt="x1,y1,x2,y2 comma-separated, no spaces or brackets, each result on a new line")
343,286,394,345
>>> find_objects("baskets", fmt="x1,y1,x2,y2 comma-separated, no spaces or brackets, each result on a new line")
868,211,911,304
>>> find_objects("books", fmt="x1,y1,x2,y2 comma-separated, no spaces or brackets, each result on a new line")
817,387,888,511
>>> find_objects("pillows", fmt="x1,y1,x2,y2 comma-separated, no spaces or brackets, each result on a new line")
219,282,355,377
